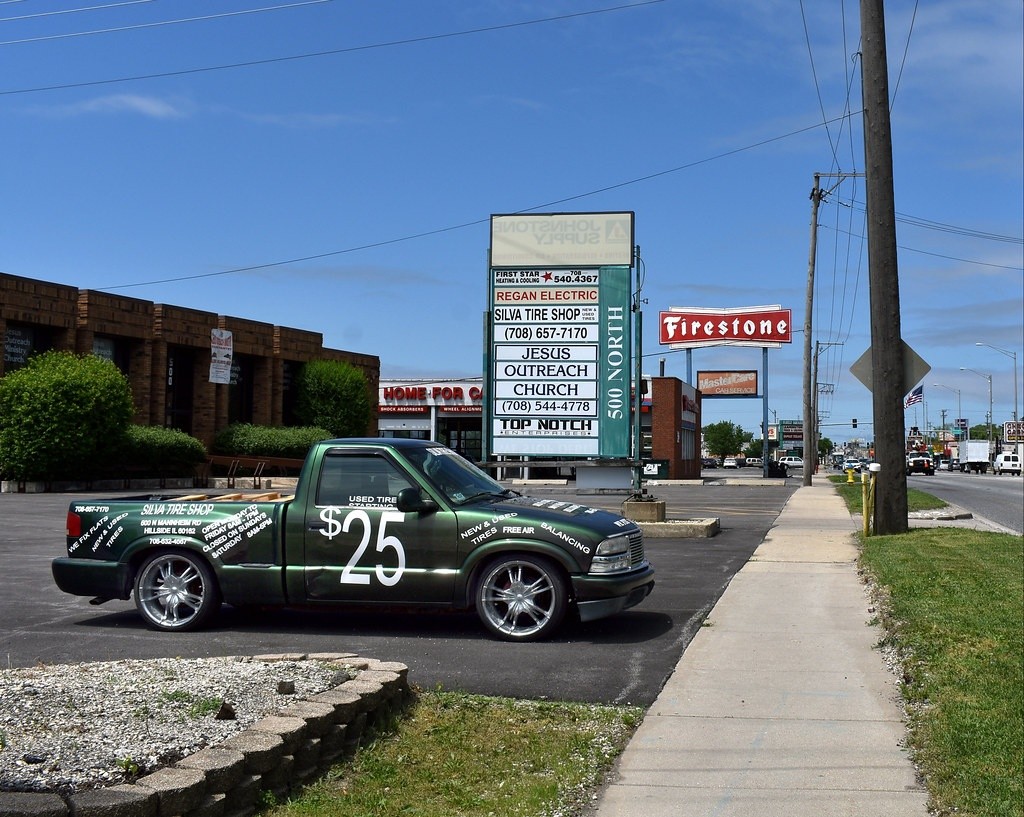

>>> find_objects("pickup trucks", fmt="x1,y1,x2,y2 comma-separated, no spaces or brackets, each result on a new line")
50,439,658,642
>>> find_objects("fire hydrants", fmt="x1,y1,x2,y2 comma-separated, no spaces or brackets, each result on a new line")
847,464,855,483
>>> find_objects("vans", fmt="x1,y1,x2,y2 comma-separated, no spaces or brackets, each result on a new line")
994,454,1022,476
745,457,764,467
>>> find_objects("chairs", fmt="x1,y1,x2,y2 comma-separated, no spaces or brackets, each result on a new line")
374,463,389,497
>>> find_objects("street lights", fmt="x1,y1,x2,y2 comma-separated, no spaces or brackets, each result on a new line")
960,367,994,470
974,342,1018,453
934,383,962,441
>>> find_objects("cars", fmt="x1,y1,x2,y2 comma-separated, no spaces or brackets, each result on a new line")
833,454,874,473
701,457,723,468
941,459,962,471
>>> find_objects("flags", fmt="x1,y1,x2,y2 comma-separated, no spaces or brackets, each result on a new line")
903,384,922,408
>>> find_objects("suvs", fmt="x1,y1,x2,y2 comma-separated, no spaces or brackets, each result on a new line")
778,456,804,469
724,457,738,469
905,452,935,475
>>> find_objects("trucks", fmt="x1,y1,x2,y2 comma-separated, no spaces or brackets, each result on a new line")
960,440,989,474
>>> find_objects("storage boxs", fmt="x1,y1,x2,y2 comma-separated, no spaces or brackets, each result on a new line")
244,492,281,502
164,494,208,502
270,495,295,503
201,493,243,501
